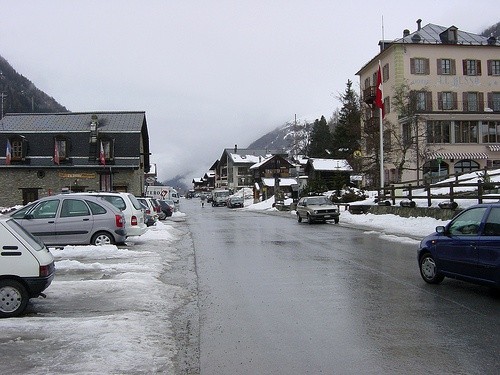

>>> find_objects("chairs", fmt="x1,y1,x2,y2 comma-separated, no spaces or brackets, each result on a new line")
60,197,112,216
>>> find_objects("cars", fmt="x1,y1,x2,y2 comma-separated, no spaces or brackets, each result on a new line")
296,195,340,225
6,195,126,249
417,204,500,292
206,188,244,208
136,185,180,227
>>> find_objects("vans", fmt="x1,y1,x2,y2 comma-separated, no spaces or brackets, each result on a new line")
0,213,55,319
70,193,147,239
